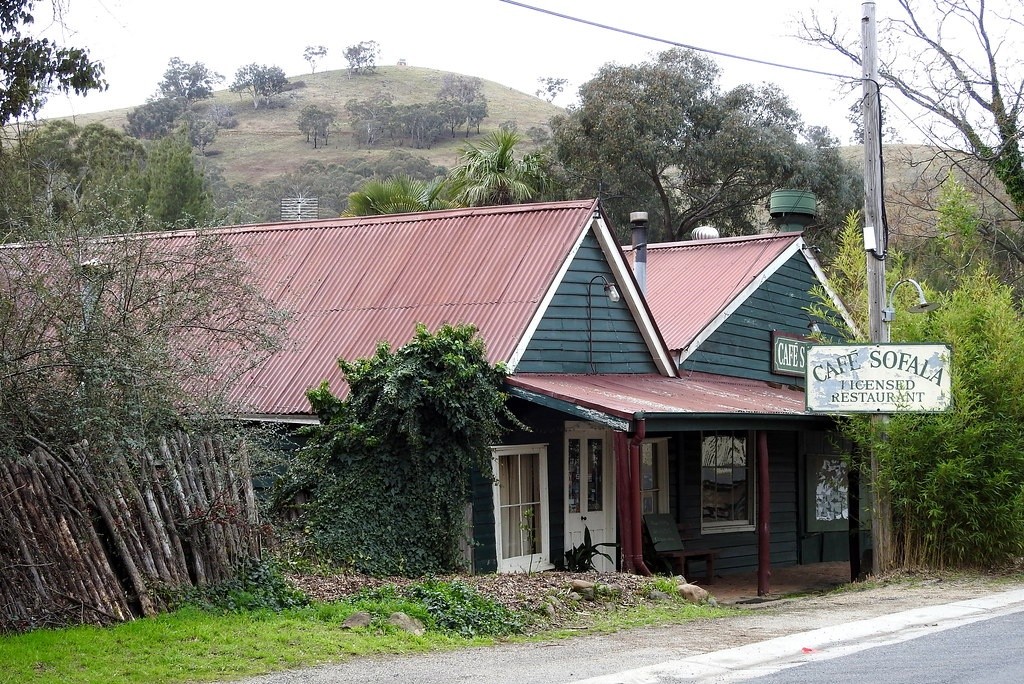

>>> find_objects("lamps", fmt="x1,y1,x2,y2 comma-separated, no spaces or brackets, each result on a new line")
799,313,821,337
589,276,620,374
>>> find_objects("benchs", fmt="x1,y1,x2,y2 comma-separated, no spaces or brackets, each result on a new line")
643,523,715,586
